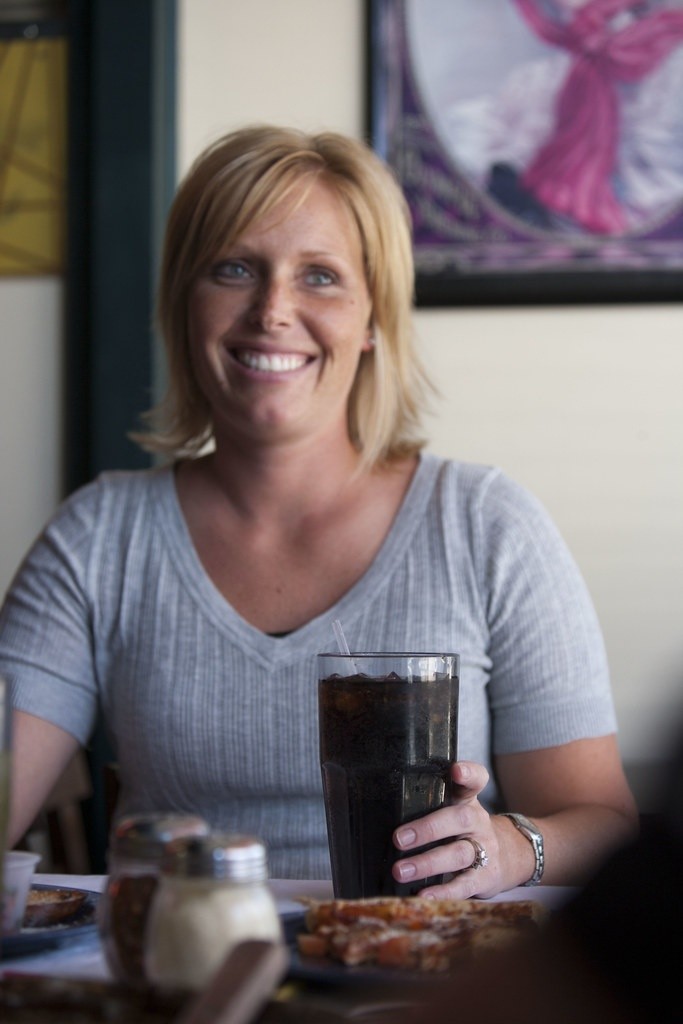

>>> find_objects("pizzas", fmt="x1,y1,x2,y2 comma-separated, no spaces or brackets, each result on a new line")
293,897,544,978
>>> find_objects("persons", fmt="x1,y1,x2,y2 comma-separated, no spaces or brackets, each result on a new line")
0,125,640,901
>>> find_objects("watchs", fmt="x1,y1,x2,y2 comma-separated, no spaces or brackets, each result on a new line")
500,813,545,886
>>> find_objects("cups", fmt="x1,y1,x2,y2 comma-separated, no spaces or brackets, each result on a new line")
0,850,41,939
316,648,462,901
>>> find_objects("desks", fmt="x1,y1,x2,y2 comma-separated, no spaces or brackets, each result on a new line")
0,874,562,1024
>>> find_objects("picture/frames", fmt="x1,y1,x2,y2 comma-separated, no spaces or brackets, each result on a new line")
362,0,683,303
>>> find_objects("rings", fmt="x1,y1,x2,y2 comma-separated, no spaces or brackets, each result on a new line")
461,837,488,870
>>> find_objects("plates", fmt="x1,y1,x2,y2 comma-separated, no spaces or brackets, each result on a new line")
0,884,103,953
277,915,412,984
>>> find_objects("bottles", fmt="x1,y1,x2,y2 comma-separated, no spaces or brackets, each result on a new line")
100,815,218,980
145,833,282,993
